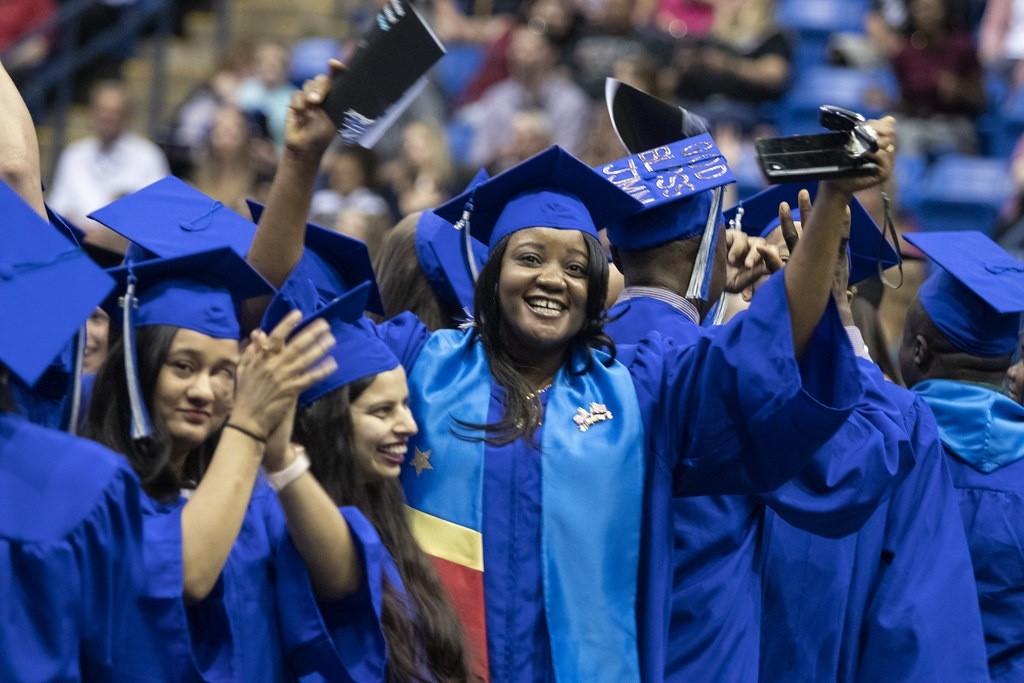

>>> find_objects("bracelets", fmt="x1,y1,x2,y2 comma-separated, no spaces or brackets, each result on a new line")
224,420,265,446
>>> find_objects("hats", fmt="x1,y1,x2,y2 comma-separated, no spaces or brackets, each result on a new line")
86,177,257,266
592,131,737,252
413,167,493,326
900,229,1023,356
245,198,387,332
434,144,648,258
0,175,117,387
286,280,402,411
723,177,903,290
107,242,278,337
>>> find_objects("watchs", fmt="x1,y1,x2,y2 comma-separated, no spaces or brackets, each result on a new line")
264,441,311,492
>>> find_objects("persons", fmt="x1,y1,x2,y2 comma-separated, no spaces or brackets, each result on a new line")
587,130,917,679
0,184,176,683
267,283,495,682
246,3,901,683
0,1,1024,421
894,224,1024,683
99,241,394,683
703,164,990,683
